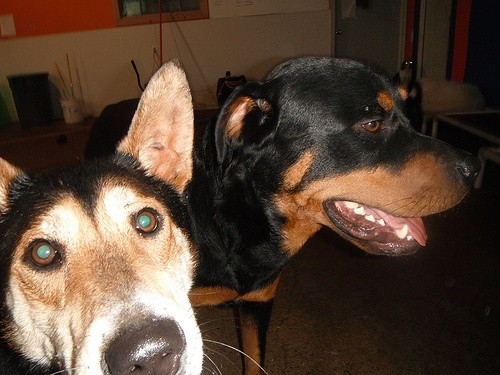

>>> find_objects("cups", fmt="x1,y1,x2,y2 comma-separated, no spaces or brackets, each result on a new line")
61,99,83,123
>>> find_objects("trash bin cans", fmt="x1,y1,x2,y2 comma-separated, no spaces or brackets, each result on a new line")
8,72,52,127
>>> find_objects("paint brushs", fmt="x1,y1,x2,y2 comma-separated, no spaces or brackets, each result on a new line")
55,56,74,100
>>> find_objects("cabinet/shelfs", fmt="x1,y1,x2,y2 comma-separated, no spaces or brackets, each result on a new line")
0,116,95,173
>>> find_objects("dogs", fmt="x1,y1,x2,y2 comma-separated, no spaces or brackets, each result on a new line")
0,57,270,375
84,55,482,374
